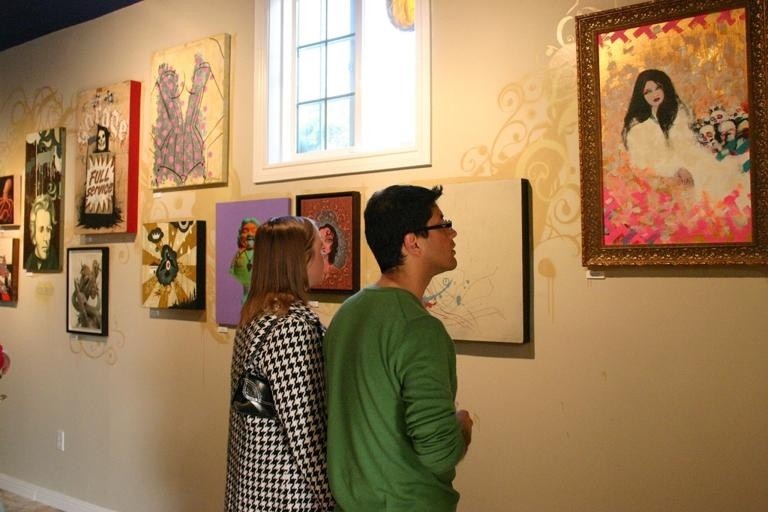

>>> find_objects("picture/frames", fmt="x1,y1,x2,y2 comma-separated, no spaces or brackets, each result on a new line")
294,190,363,294
575,4,768,271
65,247,109,335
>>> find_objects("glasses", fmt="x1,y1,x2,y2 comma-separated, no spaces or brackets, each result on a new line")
402,217,453,243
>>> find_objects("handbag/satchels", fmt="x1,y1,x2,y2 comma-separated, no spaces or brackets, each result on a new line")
232,373,278,419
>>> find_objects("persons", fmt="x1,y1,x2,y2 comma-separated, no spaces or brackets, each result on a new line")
229,218,260,304
24,195,59,272
323,184,473,512
319,225,337,272
621,70,751,210
224,218,338,511
